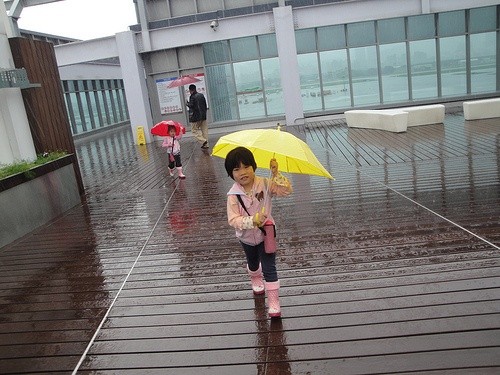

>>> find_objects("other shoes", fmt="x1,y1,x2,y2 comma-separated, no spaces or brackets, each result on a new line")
200,141,208,148
204,146,209,150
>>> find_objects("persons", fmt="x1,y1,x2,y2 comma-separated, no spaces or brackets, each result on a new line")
225,146,292,316
184,84,210,149
162,126,186,178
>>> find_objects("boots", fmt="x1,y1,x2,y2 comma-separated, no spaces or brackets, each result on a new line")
175,166,186,178
246,262,265,295
168,166,175,177
264,279,282,318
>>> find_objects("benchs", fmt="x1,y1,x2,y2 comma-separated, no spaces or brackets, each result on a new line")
344,103,445,132
463,98,500,120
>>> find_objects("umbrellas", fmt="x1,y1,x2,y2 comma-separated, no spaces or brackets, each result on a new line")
210,122,334,224
151,120,185,137
167,74,201,89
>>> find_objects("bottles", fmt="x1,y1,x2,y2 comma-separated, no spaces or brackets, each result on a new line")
263,221,276,253
